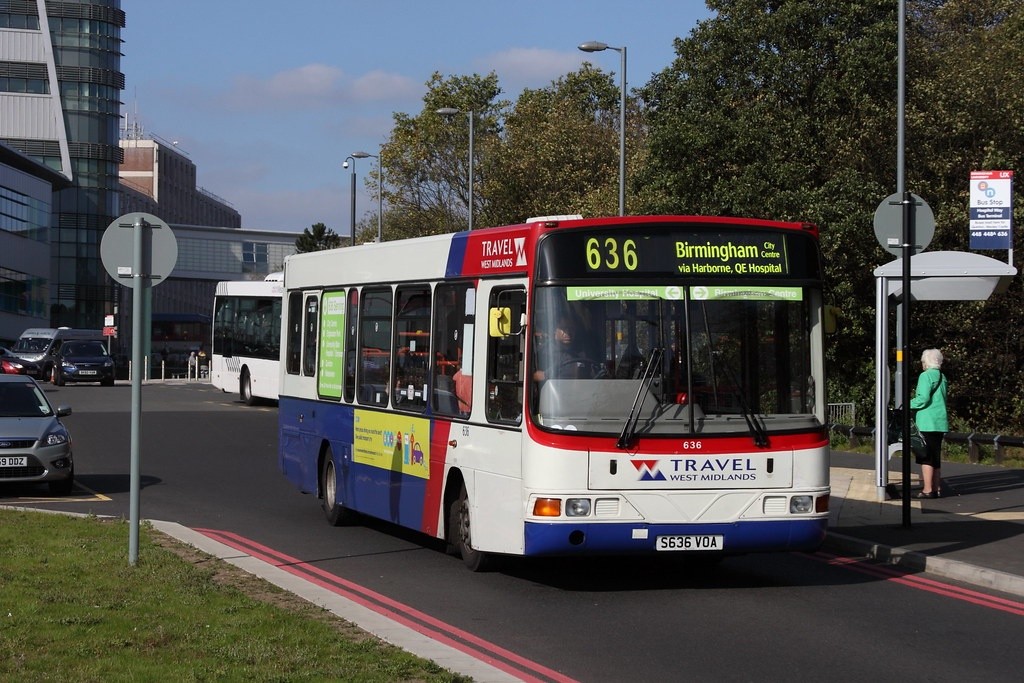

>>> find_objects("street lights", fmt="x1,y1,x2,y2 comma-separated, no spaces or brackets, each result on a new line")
436,108,473,232
342,156,356,246
577,41,628,217
352,150,383,239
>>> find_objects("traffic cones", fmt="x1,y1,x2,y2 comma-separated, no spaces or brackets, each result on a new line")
50,368,56,385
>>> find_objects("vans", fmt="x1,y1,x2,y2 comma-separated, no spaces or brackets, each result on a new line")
10,327,106,381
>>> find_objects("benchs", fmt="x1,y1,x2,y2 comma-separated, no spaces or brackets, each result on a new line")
363,349,444,392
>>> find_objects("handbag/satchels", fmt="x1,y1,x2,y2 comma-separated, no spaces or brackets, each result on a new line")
909,418,927,449
887,404,903,445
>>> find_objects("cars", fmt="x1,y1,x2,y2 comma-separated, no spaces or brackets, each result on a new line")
0,346,42,380
52,340,116,388
0,373,74,496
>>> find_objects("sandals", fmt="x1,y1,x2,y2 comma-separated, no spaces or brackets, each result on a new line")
912,490,934,498
933,490,943,497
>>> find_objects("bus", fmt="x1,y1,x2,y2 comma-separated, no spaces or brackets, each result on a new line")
150,311,212,377
279,215,844,572
211,273,280,405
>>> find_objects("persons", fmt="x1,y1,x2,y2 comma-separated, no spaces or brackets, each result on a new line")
188,350,209,379
909,349,949,499
531,315,612,391
